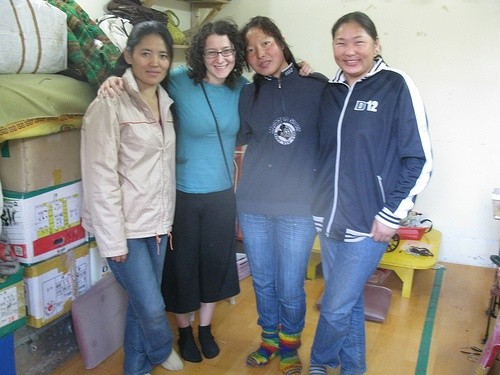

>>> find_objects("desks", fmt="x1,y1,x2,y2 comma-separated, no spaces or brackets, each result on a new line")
307,225,442,297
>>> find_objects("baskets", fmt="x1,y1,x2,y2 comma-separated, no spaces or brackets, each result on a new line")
397,227,426,240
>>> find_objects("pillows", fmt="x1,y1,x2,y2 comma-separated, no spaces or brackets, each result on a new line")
0,0,95,143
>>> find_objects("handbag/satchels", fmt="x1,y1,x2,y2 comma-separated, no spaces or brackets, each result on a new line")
164,10,185,45
107,0,167,27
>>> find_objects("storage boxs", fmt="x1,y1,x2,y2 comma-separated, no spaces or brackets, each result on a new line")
0,129,125,375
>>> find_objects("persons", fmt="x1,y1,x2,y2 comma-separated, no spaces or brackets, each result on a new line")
164,21,312,363
235,15,331,375
78,21,182,375
308,12,426,375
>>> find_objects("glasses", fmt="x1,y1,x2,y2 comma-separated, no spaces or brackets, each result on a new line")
201,48,236,59
410,245,433,257
